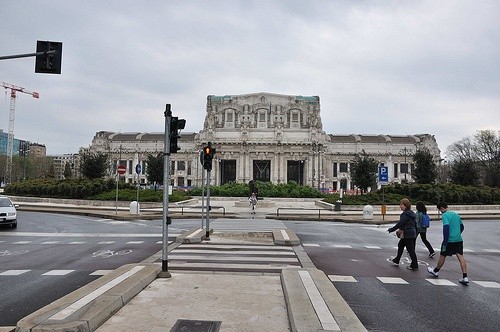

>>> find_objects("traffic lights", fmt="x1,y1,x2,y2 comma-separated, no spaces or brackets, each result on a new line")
203,146,212,169
168,117,186,154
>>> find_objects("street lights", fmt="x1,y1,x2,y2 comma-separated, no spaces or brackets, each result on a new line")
444,161,447,179
438,159,444,178
119,147,122,181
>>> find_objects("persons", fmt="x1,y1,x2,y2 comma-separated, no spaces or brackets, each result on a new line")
407,201,436,258
427,201,469,283
385,198,419,271
249,193,258,203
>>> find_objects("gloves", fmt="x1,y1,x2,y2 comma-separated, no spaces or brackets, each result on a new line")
441,245,446,252
387,228,393,233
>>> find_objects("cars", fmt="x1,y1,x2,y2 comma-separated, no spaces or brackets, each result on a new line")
0,196,19,229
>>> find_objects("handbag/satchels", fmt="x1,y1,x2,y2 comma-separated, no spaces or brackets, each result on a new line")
396,229,405,239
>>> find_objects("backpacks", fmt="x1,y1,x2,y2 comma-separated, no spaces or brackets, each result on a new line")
421,213,429,228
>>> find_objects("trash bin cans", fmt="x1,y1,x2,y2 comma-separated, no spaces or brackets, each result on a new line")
363,205,374,220
130,201,140,215
335,201,341,210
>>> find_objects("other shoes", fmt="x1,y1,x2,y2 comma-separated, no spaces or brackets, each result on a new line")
387,258,399,266
427,267,439,277
429,253,436,258
406,265,418,271
459,277,469,283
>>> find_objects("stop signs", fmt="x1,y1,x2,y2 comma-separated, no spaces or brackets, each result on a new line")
117,165,126,174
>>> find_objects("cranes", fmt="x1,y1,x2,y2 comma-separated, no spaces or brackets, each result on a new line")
0,80,40,184
